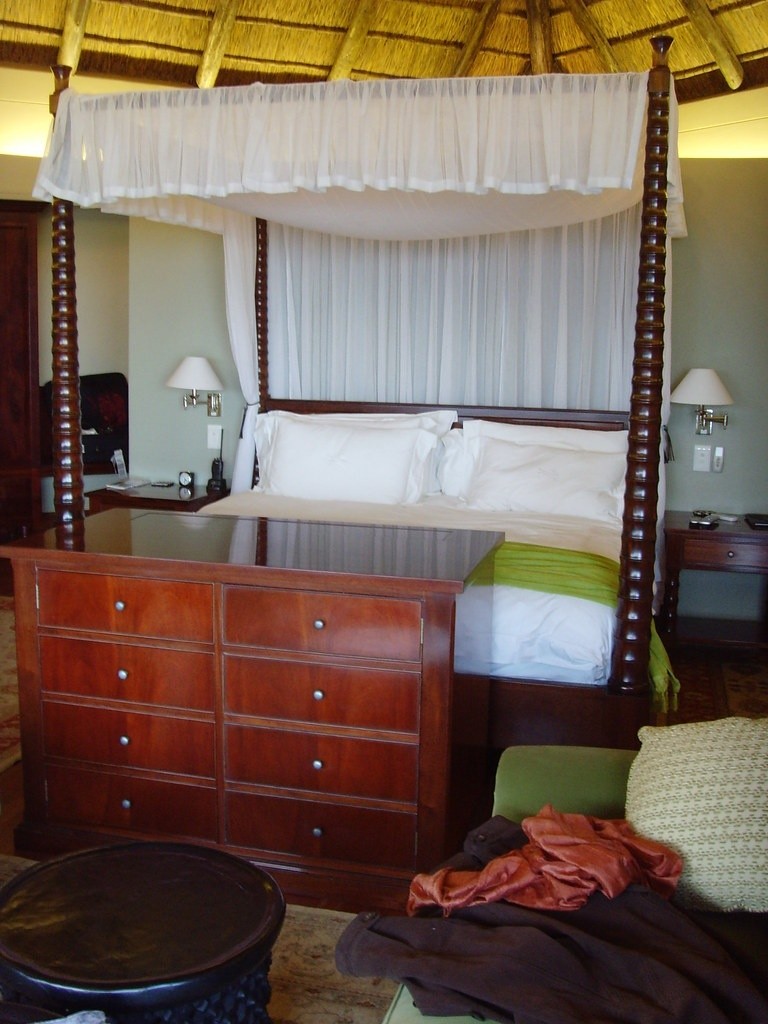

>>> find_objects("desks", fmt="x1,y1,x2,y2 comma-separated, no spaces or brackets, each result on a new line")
0,837,287,1024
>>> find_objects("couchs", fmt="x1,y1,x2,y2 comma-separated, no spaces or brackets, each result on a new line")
383,745,768,1024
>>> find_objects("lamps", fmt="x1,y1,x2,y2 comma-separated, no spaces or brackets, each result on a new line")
165,357,222,417
671,367,733,434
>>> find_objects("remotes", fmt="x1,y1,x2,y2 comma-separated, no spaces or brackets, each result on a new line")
699,515,718,525
689,513,701,523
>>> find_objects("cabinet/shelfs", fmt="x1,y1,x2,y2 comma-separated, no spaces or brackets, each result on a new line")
0,508,506,924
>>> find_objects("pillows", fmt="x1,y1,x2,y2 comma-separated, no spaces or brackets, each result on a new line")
251,408,632,528
627,716,767,913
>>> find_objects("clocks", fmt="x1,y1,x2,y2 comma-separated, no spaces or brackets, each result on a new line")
179,470,195,485
179,486,194,499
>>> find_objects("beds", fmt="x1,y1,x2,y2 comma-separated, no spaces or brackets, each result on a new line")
42,35,688,750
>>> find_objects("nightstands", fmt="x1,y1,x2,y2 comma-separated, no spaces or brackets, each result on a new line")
83,483,228,513
661,508,768,634
